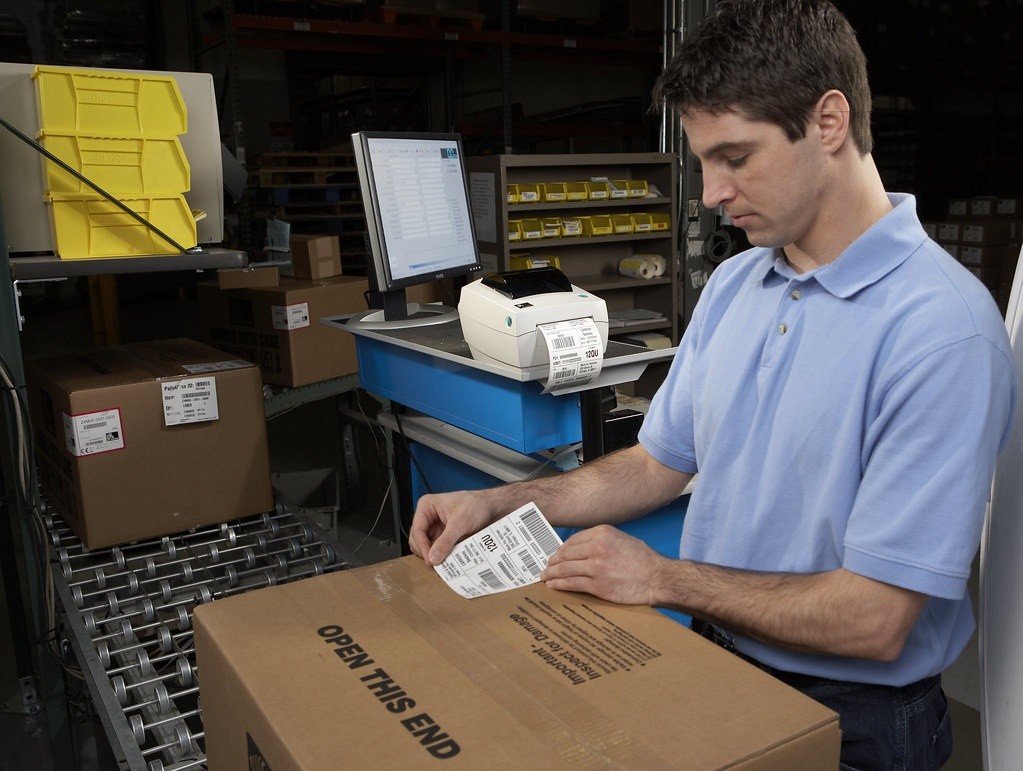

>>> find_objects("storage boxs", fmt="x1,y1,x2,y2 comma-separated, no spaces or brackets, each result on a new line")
505,179,672,269
218,267,280,291
199,276,371,389
406,285,442,304
27,337,273,552
194,553,844,771
923,197,1023,300
0,61,226,255
272,234,343,281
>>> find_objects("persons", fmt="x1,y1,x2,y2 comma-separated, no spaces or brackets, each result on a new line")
408,0,1015,771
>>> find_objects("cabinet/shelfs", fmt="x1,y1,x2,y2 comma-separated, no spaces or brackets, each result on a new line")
466,152,678,347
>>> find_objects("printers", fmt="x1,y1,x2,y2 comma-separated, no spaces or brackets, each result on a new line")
458,265,610,369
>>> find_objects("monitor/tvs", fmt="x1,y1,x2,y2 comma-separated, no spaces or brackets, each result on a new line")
345,131,483,330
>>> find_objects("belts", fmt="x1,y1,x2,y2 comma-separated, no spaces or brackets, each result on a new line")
691,619,830,688
